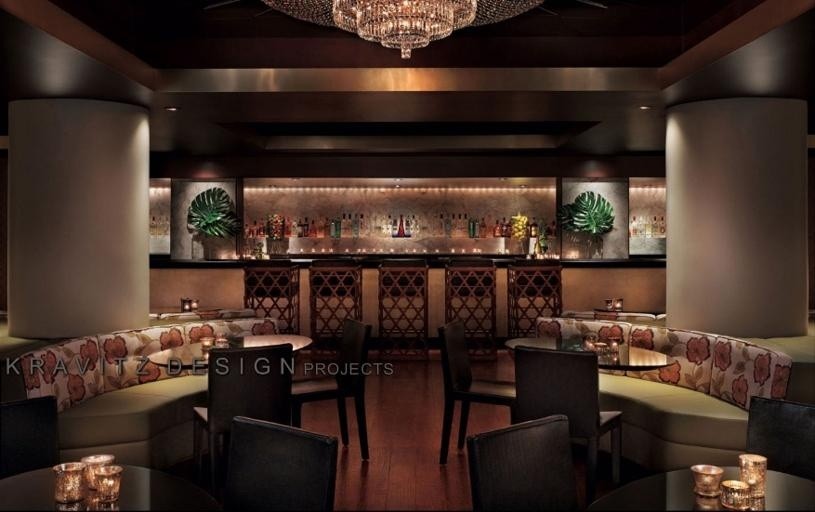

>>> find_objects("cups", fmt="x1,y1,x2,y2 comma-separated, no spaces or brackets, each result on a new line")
53,463,86,503
254,248,262,259
555,329,619,354
598,353,620,367
613,297,625,308
199,335,245,351
601,298,612,308
56,490,119,511
691,496,723,512
738,454,766,499
82,454,116,491
749,497,766,512
690,464,724,497
95,466,123,502
720,481,753,512
201,348,209,361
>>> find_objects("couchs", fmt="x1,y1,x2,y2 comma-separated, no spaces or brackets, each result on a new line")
561,309,665,326
1,317,279,470
536,315,793,473
147,307,257,326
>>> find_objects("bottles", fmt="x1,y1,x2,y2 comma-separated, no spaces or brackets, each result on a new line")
244,239,250,259
629,215,665,237
244,212,559,238
532,236,555,259
192,297,200,309
148,215,170,236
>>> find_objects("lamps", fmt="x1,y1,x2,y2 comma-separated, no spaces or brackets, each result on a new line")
263,1,545,58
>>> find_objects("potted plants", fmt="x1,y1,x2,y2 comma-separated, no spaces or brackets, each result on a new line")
186,187,241,261
553,190,615,260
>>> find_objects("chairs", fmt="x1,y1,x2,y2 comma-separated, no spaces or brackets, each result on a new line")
438,318,516,466
192,342,293,483
214,414,338,512
466,414,580,511
512,344,623,506
746,394,813,482
0,396,58,481
291,317,373,460
